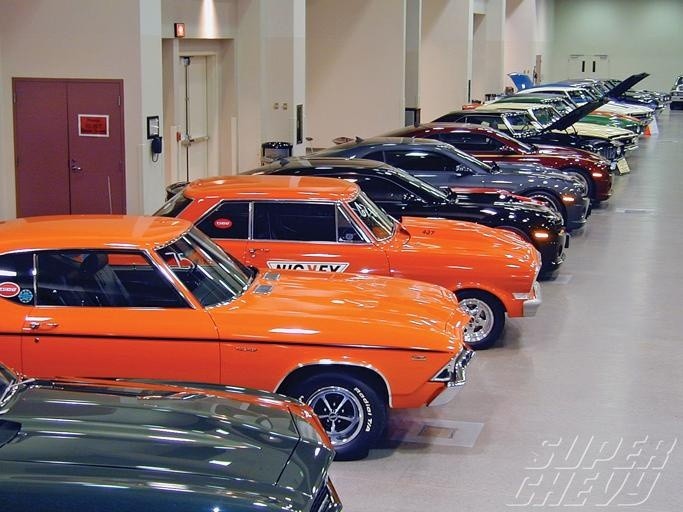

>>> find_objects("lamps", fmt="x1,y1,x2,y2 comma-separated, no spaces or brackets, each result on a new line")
174,22,185,38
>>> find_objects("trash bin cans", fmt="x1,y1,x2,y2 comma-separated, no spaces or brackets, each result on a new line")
405,107,421,128
261,142,293,167
